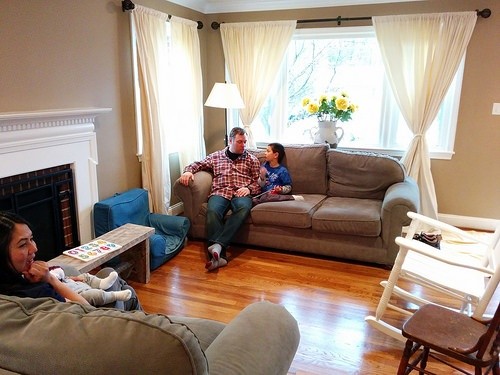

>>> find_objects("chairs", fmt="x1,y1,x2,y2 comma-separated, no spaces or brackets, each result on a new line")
365,210,500,355
396,301,500,375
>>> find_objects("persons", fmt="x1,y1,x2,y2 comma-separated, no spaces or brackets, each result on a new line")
179,127,260,271
253,143,304,207
48,267,132,308
0,209,140,311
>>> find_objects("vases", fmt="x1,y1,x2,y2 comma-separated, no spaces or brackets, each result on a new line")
318,119,344,148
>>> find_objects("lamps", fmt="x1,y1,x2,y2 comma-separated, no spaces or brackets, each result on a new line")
203,80,247,145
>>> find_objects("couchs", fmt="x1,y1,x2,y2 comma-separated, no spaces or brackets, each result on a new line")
93,188,191,271
0,295,300,375
171,143,421,269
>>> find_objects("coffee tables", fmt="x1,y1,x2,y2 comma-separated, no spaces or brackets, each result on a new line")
48,222,155,283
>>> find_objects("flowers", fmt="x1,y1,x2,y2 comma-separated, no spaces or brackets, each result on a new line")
303,92,359,123
